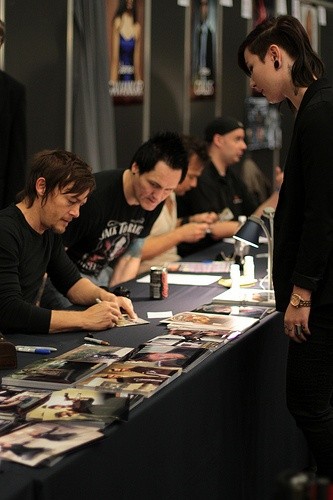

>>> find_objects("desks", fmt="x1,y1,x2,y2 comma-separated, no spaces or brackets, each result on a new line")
0,242,309,500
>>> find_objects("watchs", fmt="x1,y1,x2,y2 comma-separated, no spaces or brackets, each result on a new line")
206,229,212,239
290,294,311,308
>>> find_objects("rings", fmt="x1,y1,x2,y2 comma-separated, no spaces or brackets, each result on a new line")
297,326,302,335
288,328,293,331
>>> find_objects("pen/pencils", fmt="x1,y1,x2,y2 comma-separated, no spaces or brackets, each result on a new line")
96,298,102,303
15,345,57,353
84,337,110,346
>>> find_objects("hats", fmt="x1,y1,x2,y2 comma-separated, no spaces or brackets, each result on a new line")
203,116,244,143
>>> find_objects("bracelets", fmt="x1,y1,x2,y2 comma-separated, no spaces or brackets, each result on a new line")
182,217,189,224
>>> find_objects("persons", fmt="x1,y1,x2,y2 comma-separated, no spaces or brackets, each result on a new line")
175,116,284,257
49,393,130,419
0,149,138,336
112,366,178,376
239,15,333,482
30,426,76,439
102,374,169,385
132,348,204,368
110,0,142,96
197,0,215,94
41,126,191,309
137,138,217,273
0,441,51,459
172,329,232,339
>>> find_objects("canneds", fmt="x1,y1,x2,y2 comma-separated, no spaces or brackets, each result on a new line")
149,267,168,299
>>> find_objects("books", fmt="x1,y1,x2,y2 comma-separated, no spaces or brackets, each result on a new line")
0,289,276,468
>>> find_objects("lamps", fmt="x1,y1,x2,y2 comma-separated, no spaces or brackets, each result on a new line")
233,215,273,291
261,207,277,238
